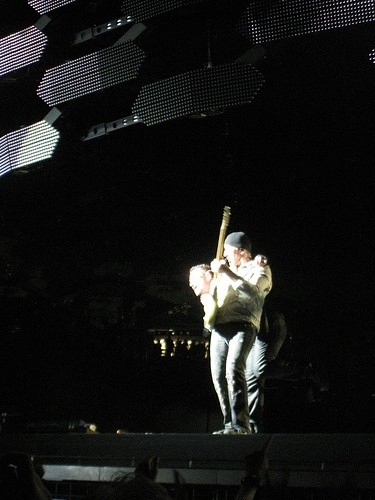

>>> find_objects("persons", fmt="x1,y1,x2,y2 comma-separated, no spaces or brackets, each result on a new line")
187,264,289,434
0,444,375,499
203,231,274,435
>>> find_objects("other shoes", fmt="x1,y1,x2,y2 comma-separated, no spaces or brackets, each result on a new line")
212,428,229,434
224,427,250,434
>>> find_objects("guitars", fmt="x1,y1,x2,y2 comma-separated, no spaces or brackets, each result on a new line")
209,206,232,302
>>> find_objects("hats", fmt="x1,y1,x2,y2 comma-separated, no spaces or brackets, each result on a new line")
225,231,252,253
